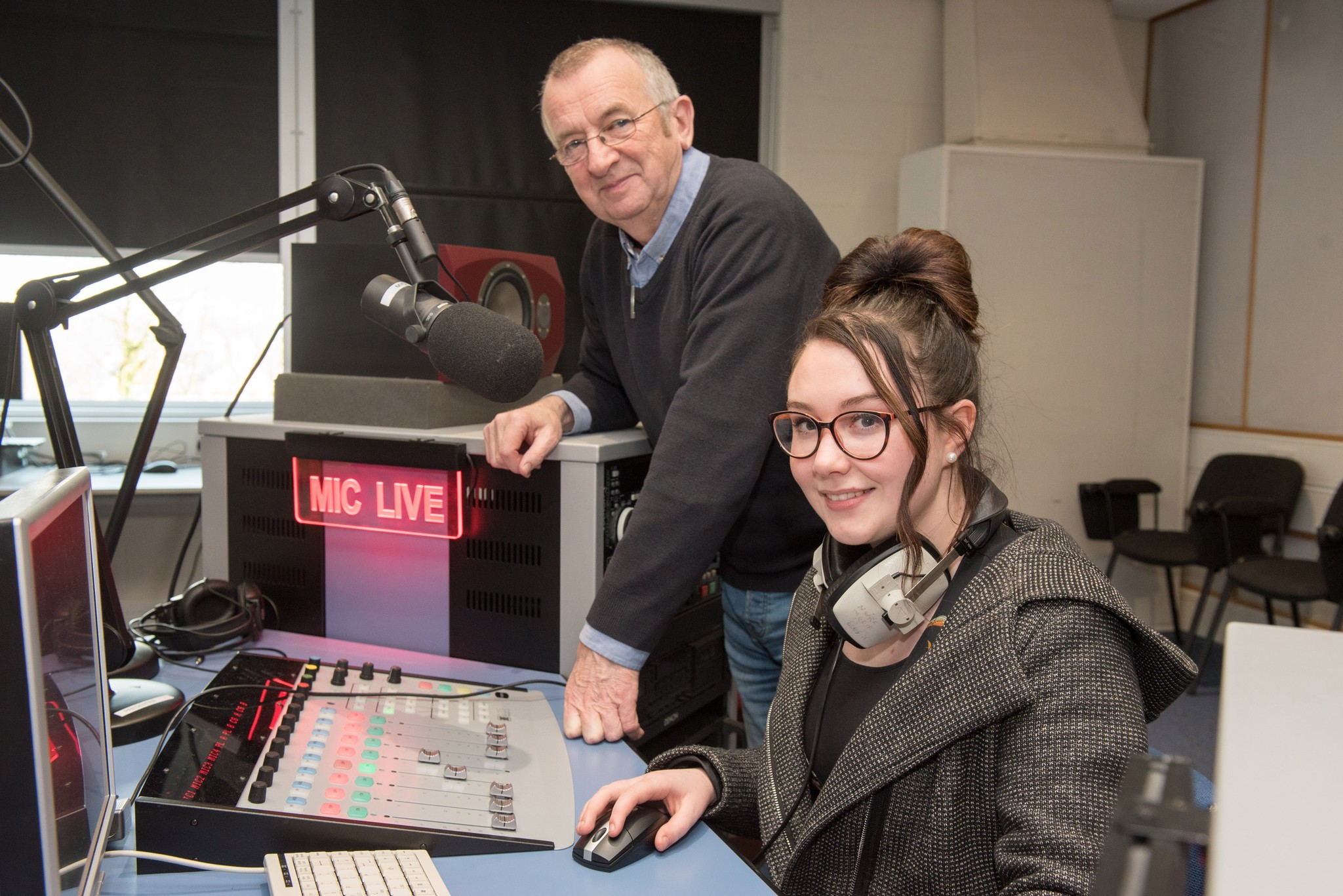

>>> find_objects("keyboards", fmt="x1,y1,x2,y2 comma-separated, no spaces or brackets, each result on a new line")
263,849,450,896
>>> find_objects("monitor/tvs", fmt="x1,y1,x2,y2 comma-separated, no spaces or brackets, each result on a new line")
2,465,116,892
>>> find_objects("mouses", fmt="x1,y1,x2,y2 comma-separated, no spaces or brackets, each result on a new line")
572,804,669,873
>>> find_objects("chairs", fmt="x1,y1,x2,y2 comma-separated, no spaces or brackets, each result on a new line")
1078,453,1342,697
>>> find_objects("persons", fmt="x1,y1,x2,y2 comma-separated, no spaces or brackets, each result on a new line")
483,36,843,747
572,230,1198,895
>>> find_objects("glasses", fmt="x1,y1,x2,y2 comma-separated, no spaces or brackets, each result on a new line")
767,400,956,461
549,101,665,166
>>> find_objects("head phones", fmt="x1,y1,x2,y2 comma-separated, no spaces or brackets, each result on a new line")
811,469,1009,650
138,579,263,657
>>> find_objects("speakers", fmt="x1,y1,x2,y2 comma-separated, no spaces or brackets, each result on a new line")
283,240,566,382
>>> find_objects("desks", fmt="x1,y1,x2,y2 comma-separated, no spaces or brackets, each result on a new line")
91,623,783,896
0,464,201,500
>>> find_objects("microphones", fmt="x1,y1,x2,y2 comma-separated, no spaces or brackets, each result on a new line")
360,275,543,404
